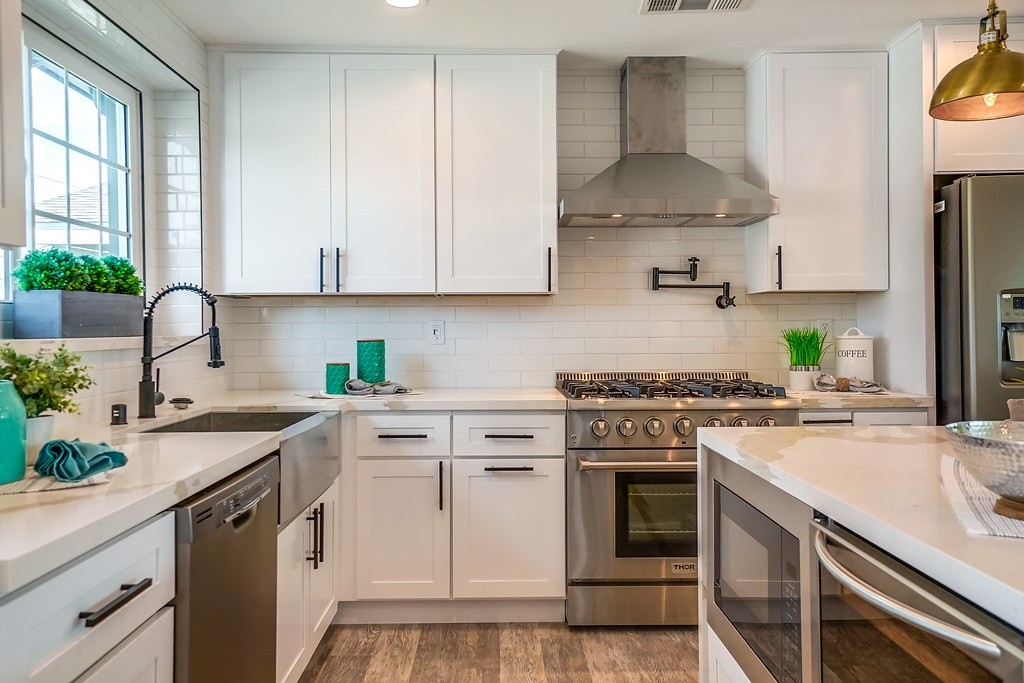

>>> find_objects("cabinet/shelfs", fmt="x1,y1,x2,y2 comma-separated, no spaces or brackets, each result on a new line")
342,411,566,626
0,512,175,683
203,45,561,298
277,480,339,683
742,46,890,293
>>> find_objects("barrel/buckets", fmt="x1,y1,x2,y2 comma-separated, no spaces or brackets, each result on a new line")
835,328,874,383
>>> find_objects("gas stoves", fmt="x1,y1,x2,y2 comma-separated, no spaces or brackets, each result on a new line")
554,370,801,447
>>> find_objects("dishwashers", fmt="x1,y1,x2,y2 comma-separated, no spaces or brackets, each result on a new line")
564,442,706,628
174,454,285,682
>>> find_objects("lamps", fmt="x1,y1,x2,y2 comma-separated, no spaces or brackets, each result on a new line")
929,0,1024,121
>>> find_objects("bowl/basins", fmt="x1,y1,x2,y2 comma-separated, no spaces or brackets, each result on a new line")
944,421,1024,503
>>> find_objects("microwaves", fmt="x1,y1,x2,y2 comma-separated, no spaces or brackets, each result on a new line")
700,447,808,683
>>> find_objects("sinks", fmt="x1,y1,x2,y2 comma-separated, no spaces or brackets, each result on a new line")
136,404,321,434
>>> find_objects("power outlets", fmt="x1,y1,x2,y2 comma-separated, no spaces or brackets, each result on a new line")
818,318,832,343
428,321,445,345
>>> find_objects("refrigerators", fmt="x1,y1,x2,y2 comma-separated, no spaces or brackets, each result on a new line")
936,172,1024,429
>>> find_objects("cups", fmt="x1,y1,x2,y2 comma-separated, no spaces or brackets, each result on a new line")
326,362,349,395
357,339,385,383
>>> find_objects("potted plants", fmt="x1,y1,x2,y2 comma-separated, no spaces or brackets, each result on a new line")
777,326,832,391
0,342,96,468
11,245,144,338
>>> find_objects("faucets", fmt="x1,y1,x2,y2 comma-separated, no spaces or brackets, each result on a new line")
137,281,226,420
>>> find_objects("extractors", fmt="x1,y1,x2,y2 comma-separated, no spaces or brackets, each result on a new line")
558,57,780,228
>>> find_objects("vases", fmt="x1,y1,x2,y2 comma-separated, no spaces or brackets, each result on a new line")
0,380,28,484
